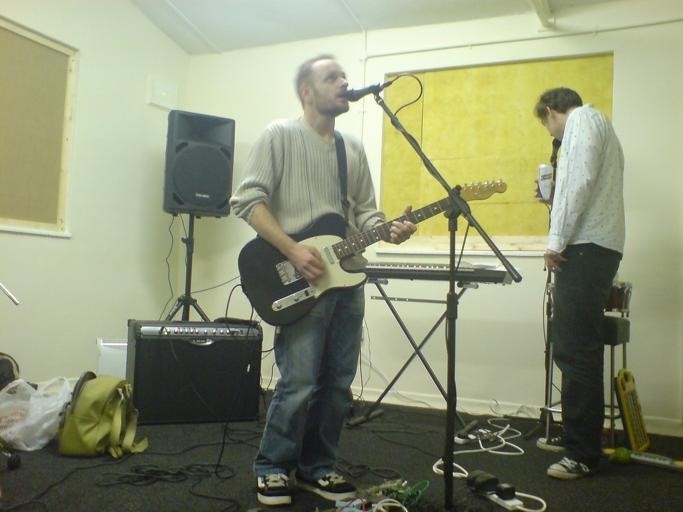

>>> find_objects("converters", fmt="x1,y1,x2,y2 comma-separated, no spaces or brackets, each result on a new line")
466,469,498,496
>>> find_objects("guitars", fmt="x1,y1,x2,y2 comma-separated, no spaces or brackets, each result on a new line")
238,178,508,327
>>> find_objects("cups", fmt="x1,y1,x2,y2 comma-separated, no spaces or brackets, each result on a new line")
538,164,554,201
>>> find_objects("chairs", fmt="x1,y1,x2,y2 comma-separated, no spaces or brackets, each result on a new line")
540,277,633,450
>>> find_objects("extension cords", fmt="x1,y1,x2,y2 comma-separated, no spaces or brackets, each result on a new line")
485,488,524,511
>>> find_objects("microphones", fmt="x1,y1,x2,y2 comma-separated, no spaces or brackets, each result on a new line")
346,82,391,102
550,138,561,168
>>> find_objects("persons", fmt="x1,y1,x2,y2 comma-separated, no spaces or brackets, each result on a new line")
536,85,625,482
229,53,417,507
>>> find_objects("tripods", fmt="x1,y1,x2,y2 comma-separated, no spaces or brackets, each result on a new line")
165,213,210,321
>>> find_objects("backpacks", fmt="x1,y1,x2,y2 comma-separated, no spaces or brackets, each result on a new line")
55,370,150,459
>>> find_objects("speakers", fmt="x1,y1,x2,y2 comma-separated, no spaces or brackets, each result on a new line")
126,318,263,424
163,110,235,218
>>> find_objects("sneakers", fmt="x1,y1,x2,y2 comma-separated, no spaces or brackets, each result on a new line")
536,435,567,453
546,456,592,481
294,468,358,502
256,472,292,506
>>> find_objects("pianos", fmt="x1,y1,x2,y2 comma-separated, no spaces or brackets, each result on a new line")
361,260,514,283
